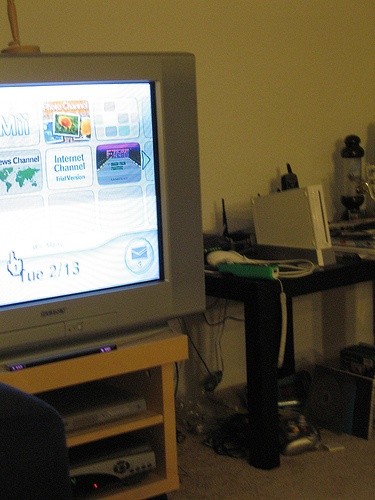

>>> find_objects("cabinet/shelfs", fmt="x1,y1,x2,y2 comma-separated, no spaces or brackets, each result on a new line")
0,333,189,500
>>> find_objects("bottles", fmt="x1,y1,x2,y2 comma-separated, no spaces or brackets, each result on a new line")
341,134,364,217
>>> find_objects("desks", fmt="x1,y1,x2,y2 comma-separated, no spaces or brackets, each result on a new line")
204,259,375,470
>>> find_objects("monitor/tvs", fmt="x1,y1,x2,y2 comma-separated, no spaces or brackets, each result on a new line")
1,53,207,358
